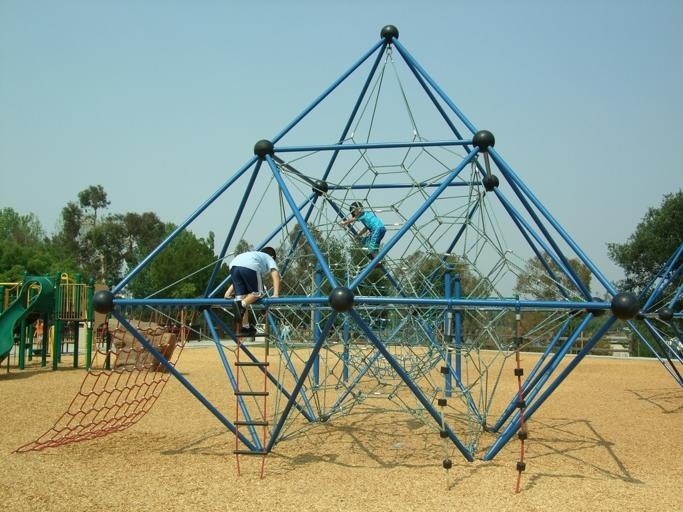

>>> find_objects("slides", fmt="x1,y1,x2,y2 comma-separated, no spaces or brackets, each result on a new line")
0,297,34,363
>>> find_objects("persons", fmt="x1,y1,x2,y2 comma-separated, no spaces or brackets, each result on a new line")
283,319,291,341
224,247,280,334
34,319,44,350
339,201,386,268
